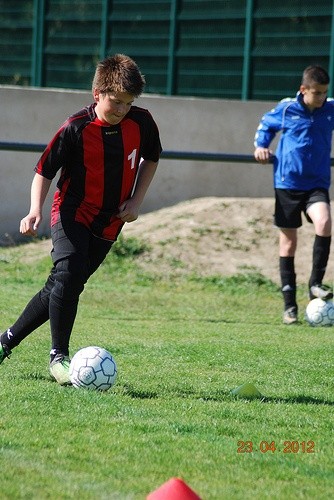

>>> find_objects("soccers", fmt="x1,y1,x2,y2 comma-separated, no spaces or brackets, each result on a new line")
68,346,118,391
305,298,334,327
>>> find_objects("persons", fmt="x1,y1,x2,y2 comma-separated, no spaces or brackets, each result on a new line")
0,53,163,386
253,65,334,325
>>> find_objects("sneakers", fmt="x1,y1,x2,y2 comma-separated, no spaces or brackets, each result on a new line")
0,341,12,366
49,351,72,385
310,283,333,300
282,305,298,324
